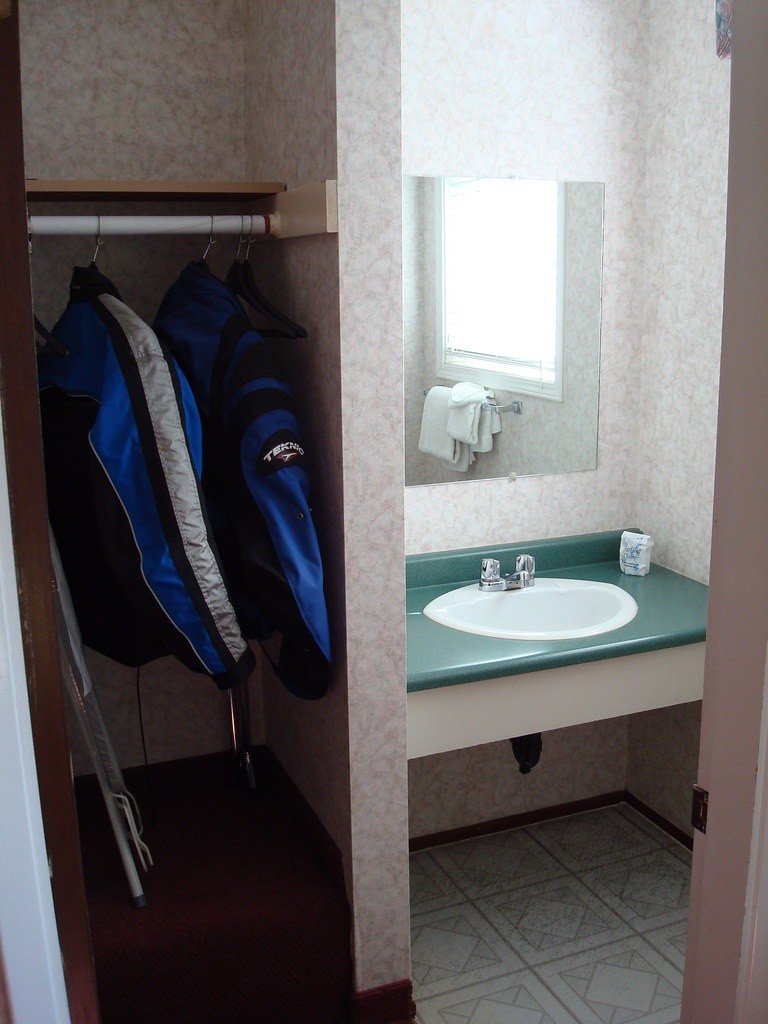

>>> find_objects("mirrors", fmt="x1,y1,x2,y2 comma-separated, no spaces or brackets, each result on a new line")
402,174,604,489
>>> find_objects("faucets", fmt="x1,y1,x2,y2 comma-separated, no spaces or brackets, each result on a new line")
504,553,535,591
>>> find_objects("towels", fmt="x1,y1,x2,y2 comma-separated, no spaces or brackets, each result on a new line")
418,381,502,472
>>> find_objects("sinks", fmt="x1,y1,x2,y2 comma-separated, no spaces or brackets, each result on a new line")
421,581,640,642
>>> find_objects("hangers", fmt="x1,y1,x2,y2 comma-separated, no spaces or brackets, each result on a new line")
83,214,253,269
